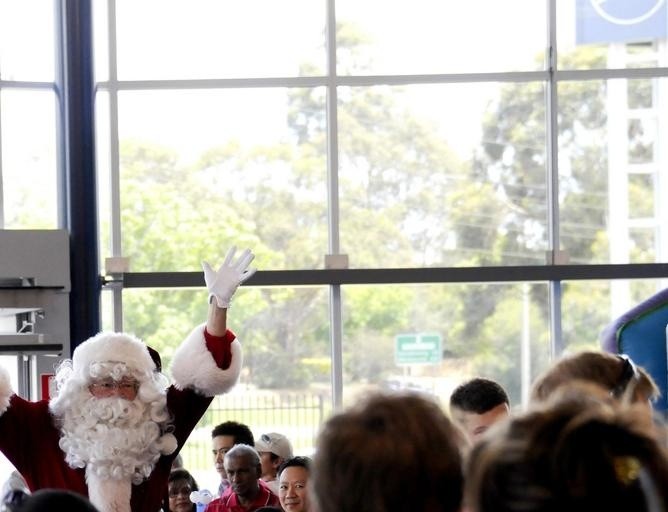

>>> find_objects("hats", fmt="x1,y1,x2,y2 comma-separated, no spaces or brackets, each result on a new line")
254,432,294,459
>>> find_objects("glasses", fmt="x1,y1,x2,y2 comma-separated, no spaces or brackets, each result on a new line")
616,352,641,409
260,433,282,450
89,381,139,394
169,486,194,499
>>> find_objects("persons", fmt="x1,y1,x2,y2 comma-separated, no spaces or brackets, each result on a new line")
0,243,258,512
2,488,100,512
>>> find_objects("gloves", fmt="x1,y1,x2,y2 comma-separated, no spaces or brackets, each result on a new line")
201,245,259,310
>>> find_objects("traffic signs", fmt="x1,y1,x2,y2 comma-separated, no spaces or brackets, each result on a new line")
394,332,444,365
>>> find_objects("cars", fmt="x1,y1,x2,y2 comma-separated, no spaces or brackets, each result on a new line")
385,373,432,394
